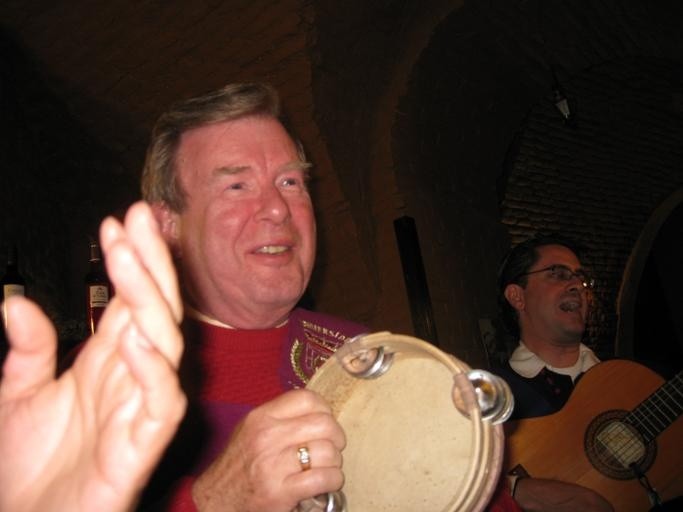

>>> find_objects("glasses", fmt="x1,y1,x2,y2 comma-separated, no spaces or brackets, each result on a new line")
518,264,594,288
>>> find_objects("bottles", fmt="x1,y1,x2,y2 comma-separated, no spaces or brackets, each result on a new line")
85,239,112,337
0,247,27,353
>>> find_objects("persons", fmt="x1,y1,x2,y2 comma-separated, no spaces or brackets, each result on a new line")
0,200,188,512
132,79,528,511
488,238,682,511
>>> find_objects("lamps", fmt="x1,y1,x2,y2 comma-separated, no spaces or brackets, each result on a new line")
551,66,577,128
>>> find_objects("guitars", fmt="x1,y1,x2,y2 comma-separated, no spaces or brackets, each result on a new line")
485,356,682,511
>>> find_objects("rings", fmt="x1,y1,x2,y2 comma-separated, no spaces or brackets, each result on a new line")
297,443,311,470
